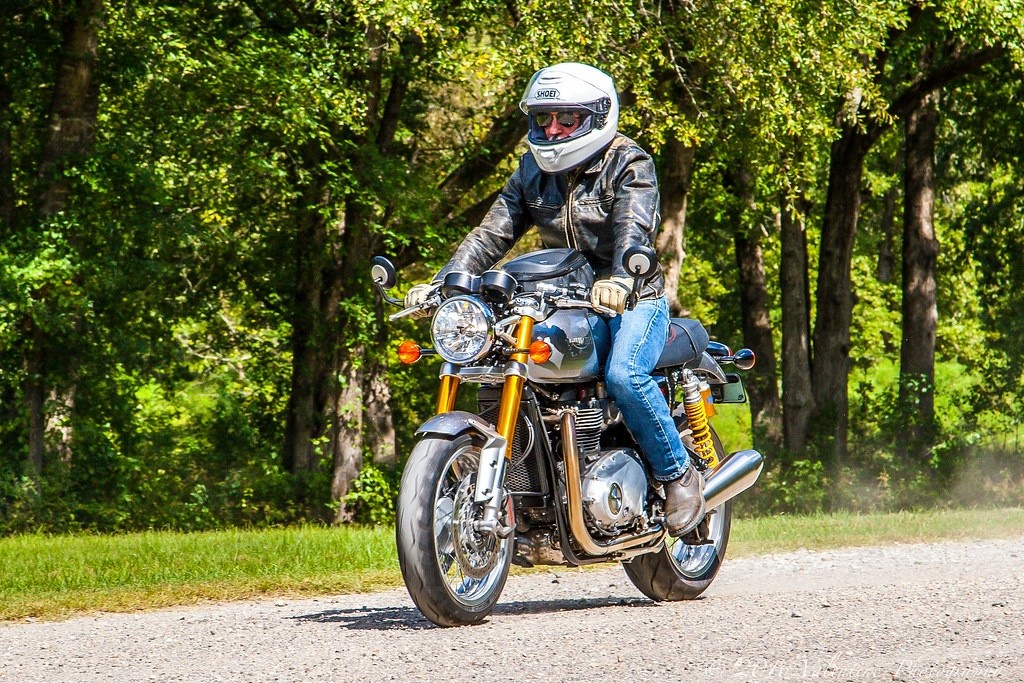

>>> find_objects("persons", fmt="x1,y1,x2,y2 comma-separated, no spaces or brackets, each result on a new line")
403,62,705,537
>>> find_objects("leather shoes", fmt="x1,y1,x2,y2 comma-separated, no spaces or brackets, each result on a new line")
664,463,705,538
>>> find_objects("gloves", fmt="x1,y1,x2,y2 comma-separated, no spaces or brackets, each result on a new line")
589,276,634,314
404,284,439,320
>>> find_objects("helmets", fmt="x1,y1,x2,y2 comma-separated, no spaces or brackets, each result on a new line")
520,63,619,172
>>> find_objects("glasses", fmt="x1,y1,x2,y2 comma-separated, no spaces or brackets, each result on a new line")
534,113,581,128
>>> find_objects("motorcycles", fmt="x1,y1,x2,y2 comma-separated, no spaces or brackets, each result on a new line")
368,255,767,630
